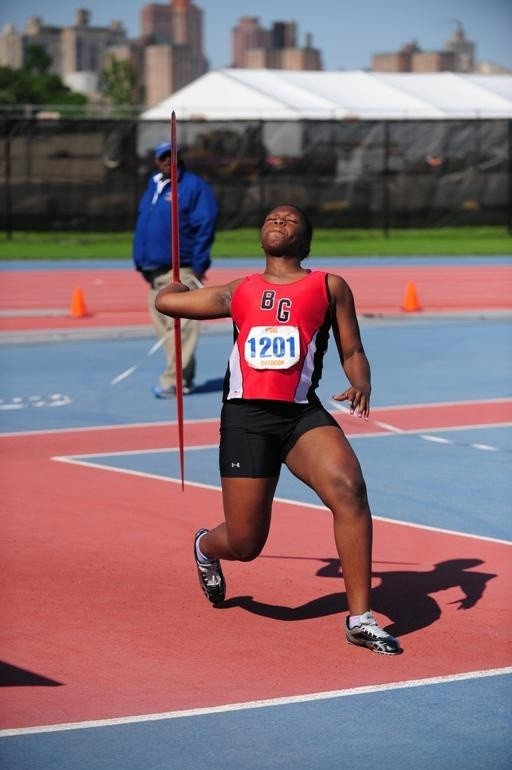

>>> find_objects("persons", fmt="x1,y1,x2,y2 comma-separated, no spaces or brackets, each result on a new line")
154,202,402,654
132,139,223,399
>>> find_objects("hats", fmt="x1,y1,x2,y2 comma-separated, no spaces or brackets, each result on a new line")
153,141,181,159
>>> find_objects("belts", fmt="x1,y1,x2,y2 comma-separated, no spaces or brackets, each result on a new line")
135,262,192,289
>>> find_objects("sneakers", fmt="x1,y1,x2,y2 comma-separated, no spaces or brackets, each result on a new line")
344,611,401,655
193,528,225,604
154,381,197,399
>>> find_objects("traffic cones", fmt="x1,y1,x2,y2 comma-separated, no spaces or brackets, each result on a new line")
70,286,92,319
401,283,421,311
